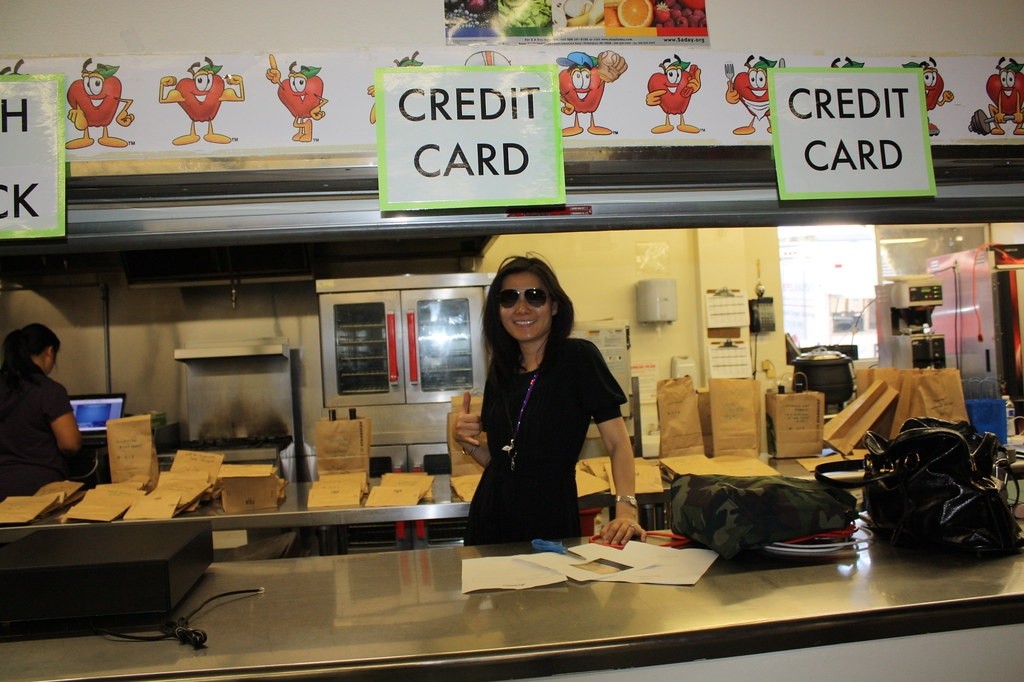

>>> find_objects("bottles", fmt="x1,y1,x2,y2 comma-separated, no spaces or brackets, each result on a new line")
1001,395,1015,437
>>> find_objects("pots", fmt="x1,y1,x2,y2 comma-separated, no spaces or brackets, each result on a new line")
790,347,856,404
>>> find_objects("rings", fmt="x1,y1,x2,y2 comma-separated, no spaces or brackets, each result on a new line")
632,525,636,530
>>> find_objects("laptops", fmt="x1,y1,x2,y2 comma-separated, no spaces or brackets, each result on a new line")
68,393,126,445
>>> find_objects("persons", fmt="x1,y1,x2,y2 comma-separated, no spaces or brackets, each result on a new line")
0,323,82,501
452,250,646,544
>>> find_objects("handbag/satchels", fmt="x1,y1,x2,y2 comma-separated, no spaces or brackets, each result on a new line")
765,374,824,457
822,367,971,454
813,414,1020,559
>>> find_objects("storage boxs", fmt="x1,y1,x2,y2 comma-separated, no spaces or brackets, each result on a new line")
218,464,278,513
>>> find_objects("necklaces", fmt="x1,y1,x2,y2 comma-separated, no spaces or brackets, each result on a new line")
502,375,537,470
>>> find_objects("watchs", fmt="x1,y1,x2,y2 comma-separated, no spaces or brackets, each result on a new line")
616,495,637,507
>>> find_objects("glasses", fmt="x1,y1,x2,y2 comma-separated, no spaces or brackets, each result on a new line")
496,287,555,308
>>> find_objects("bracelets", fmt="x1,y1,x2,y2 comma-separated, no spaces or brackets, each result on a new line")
462,447,475,455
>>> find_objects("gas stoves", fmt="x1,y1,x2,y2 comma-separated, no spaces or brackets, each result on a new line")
157,435,293,466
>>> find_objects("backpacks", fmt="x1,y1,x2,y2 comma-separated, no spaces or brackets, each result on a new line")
671,472,858,554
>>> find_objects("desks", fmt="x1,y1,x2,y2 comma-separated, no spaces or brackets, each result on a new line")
0,457,1024,682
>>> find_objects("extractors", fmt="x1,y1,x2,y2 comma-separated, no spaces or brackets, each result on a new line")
174,336,290,360
